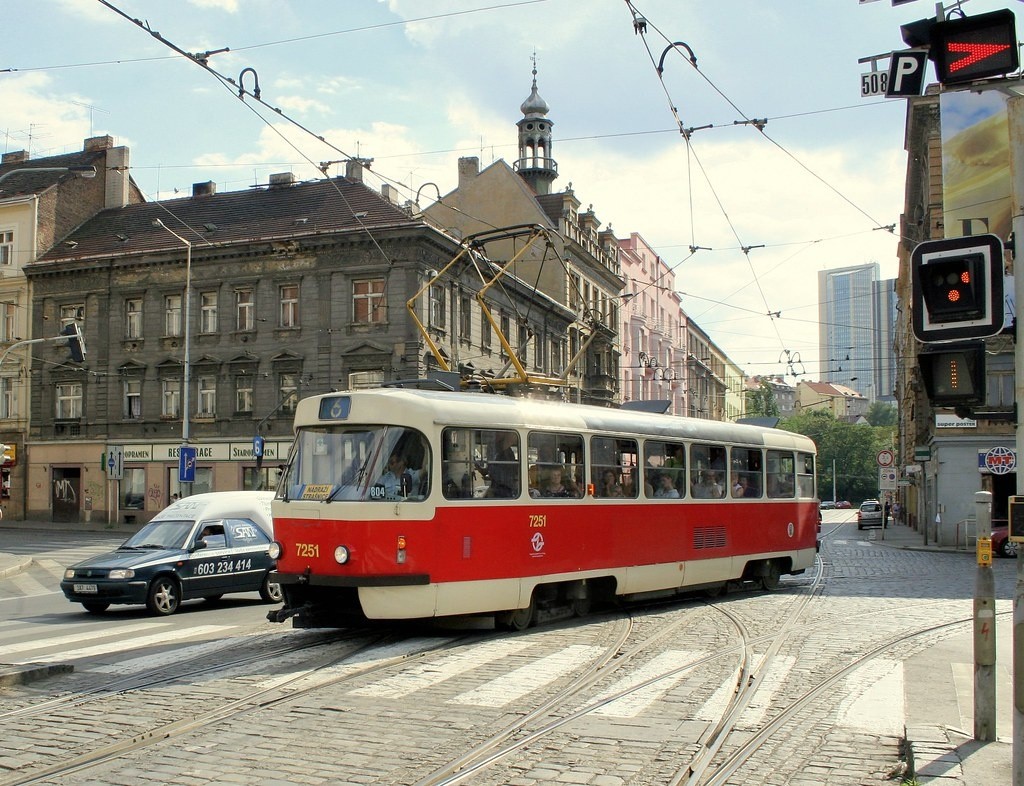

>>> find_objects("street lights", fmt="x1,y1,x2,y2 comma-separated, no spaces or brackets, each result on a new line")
152,217,193,498
714,388,750,422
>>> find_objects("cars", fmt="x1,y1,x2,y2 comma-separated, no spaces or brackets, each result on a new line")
836,501,852,510
990,525,1019,559
820,501,836,509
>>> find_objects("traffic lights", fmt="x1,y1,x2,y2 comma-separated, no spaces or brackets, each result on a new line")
926,7,1020,86
58,323,88,362
1007,495,1024,544
908,233,1006,345
916,341,988,407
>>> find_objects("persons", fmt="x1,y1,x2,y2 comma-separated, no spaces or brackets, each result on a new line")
173,493,181,503
198,528,214,540
376,450,429,497
535,442,804,499
471,439,518,498
885,501,904,526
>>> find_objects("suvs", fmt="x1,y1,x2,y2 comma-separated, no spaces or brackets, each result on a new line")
855,498,888,531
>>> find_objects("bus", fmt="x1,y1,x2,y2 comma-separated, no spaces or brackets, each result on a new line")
266,370,824,635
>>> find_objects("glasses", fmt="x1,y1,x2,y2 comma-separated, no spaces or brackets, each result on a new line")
390,462,396,465
202,534,209,536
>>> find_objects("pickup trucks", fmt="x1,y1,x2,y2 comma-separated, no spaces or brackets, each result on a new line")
58,487,285,616
